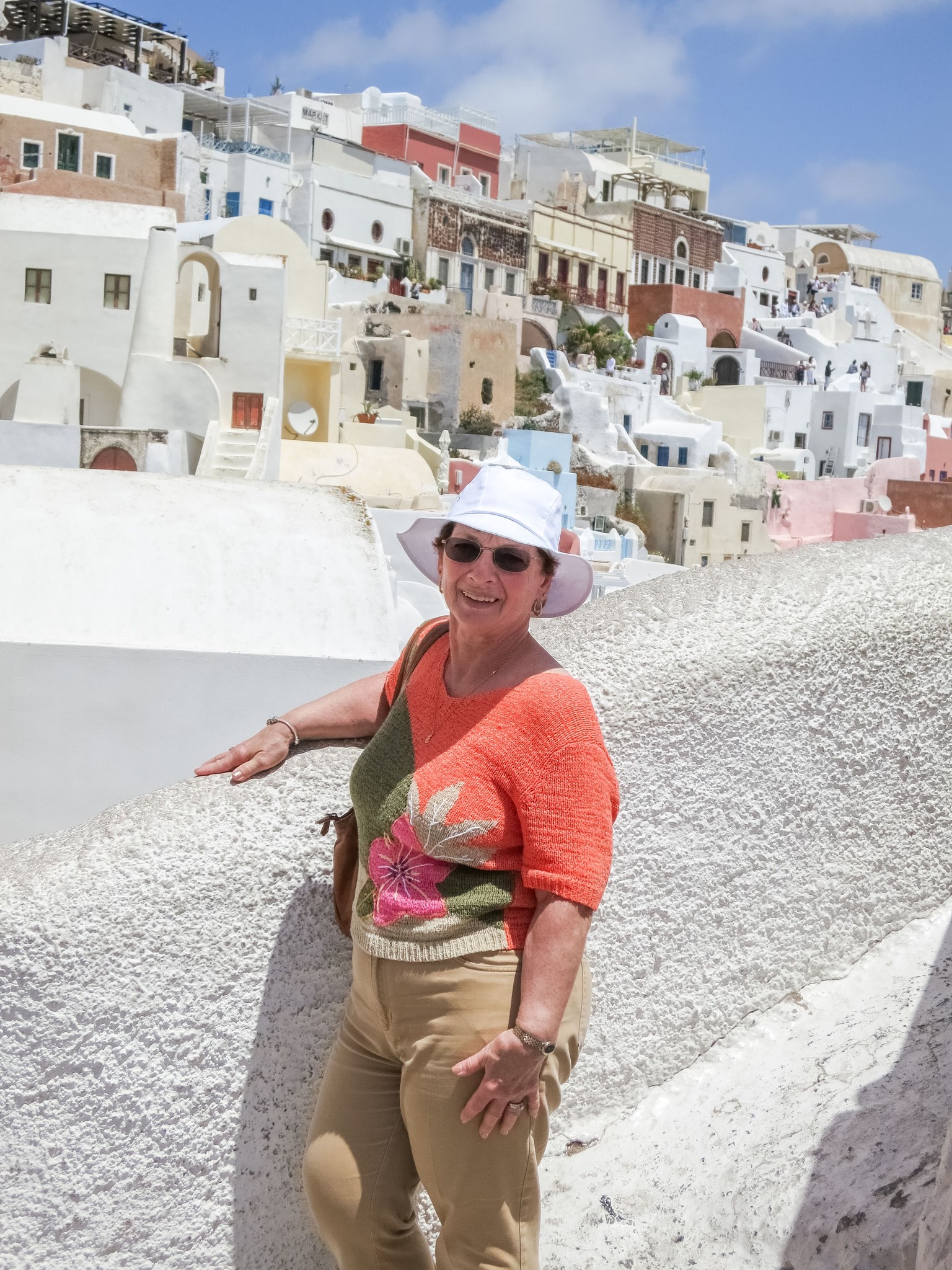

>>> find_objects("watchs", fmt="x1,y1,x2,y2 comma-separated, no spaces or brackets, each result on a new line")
513,1024,557,1055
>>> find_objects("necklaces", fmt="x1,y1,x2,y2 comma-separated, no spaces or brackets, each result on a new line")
425,633,530,743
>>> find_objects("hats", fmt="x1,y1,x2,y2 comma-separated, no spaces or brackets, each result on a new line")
396,466,594,618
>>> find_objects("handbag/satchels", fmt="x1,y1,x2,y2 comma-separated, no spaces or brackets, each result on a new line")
315,806,363,939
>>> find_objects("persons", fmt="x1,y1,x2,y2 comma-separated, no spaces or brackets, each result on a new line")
194,465,620,1269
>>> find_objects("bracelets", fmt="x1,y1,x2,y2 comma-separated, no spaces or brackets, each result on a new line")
266,716,300,745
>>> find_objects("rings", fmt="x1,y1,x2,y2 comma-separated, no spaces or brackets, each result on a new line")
229,747,233,751
508,1098,524,1110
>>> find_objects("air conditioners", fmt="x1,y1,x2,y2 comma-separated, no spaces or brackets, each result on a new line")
396,237,413,256
769,430,783,442
860,498,878,514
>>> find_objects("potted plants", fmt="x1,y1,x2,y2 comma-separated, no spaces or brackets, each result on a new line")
332,264,442,293
193,59,215,83
268,75,284,95
350,394,381,424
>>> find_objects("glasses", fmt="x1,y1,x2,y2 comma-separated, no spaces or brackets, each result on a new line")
442,537,544,573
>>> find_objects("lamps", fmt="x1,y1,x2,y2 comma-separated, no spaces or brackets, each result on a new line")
455,470,462,486
